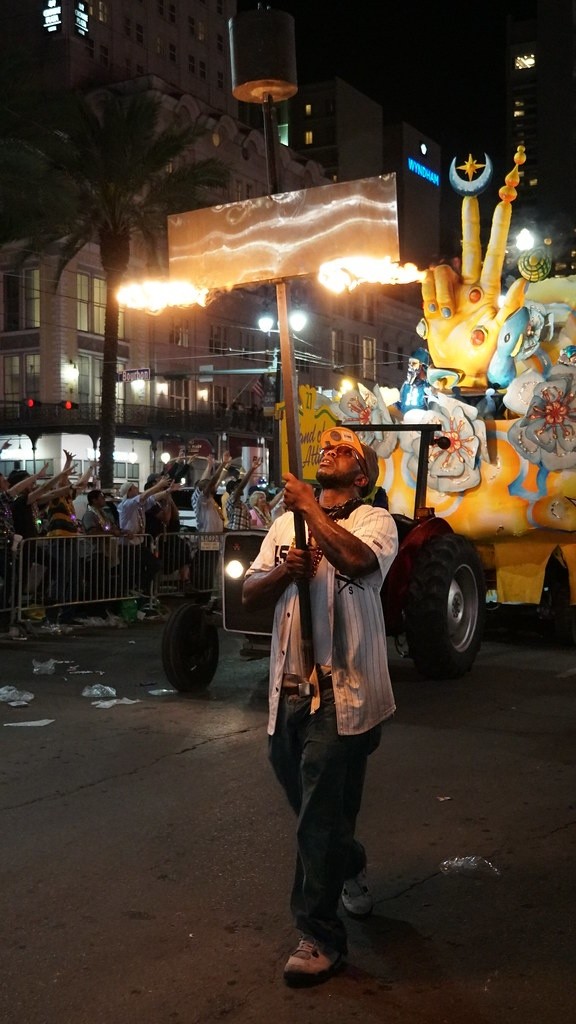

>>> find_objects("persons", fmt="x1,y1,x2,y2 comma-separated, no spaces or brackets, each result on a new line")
117,447,197,621
248,482,286,530
0,450,104,636
222,455,263,529
242,424,399,980
190,454,232,597
81,491,134,619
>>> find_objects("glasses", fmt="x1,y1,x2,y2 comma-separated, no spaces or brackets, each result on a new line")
321,445,369,478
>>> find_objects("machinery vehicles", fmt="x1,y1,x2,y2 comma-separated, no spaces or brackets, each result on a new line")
158,414,569,693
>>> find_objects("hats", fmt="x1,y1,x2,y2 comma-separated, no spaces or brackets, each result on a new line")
323,426,380,497
120,482,133,503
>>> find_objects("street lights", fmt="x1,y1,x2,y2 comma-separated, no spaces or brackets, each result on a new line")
255,309,308,404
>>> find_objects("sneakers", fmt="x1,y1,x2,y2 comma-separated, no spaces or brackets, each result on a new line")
342,848,374,916
284,932,348,985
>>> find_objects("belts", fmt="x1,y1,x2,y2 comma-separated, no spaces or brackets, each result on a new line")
280,677,332,698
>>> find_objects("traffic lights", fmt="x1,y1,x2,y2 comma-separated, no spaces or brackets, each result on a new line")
21,398,41,407
60,399,80,410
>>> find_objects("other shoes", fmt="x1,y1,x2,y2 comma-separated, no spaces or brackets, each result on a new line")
183,578,196,592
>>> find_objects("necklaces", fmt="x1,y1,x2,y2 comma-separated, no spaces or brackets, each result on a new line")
306,491,361,580
